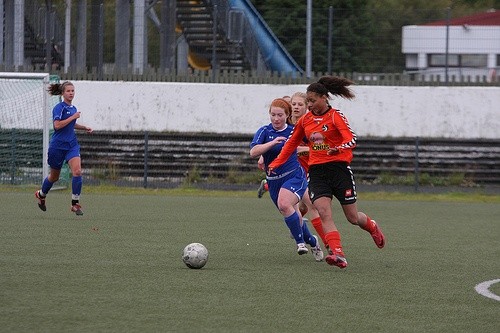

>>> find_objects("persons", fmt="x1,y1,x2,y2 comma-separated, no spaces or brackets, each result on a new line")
258,95,290,198
290,92,336,265
269,76,386,269
34,81,93,216
250,98,324,262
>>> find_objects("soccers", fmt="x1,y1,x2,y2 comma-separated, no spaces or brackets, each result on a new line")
182,242,209,270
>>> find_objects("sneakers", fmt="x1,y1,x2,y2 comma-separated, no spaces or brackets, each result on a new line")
296,243,309,256
309,235,324,261
369,219,385,249
34,190,47,211
325,253,347,268
256,179,268,198
71,204,83,215
